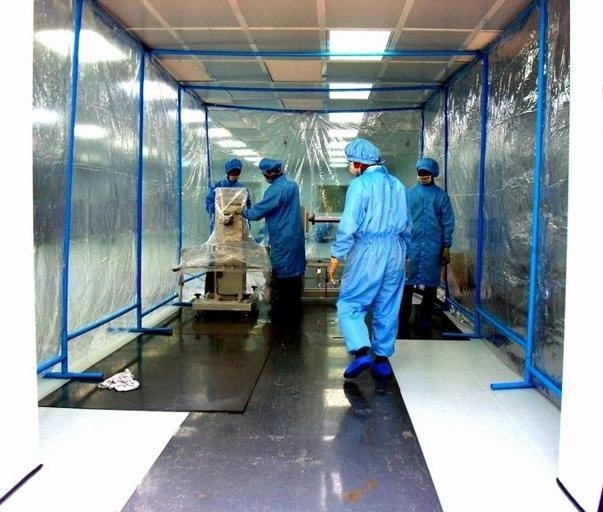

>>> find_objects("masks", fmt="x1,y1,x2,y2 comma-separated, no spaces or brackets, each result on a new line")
349,162,362,176
416,175,432,184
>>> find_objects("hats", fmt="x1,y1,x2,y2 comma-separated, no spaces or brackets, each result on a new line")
225,158,243,173
344,138,386,165
416,157,439,177
259,159,281,170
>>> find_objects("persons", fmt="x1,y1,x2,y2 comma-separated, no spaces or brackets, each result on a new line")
234,157,307,339
326,138,413,380
202,158,252,296
398,156,456,334
